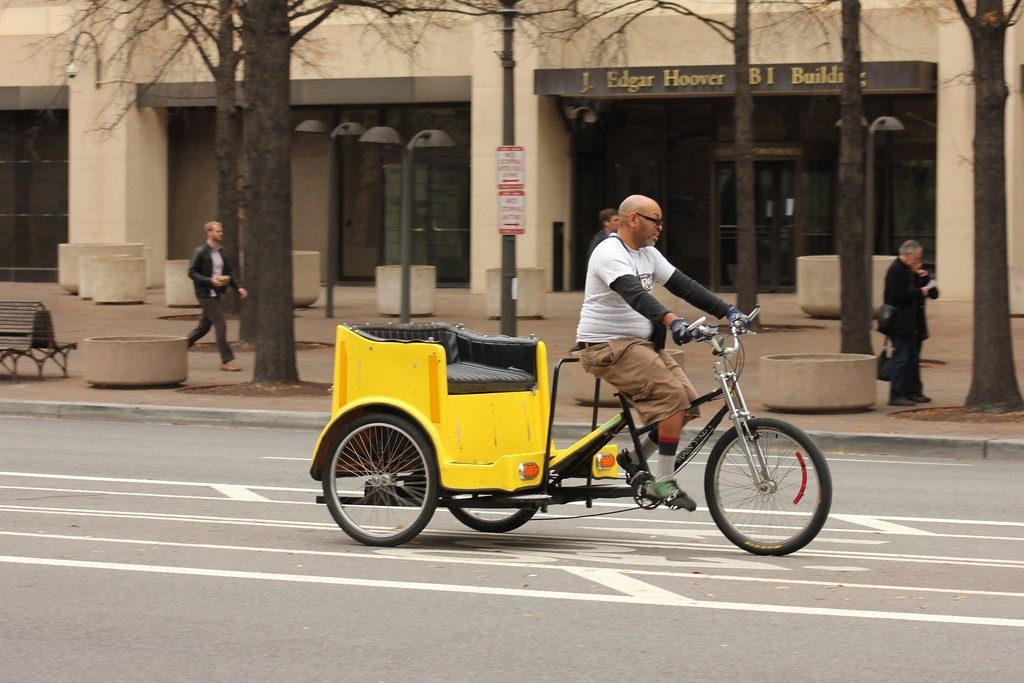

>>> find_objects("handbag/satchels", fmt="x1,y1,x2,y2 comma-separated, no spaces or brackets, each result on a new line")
875,334,893,381
873,302,897,325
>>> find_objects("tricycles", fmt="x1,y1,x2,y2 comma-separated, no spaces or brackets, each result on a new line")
307,301,832,558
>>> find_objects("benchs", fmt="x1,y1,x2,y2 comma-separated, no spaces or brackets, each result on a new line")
0,301,79,383
339,321,548,396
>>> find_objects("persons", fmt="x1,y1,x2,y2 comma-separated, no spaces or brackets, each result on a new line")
575,195,748,512
186,221,248,372
877,240,933,404
589,209,620,259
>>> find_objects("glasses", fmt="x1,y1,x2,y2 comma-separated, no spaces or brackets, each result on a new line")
635,212,664,226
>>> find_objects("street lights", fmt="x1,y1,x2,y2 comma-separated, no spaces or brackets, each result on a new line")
293,119,366,320
356,125,456,322
832,116,908,328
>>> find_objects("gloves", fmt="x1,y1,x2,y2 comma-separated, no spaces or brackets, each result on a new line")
726,303,751,335
669,318,693,346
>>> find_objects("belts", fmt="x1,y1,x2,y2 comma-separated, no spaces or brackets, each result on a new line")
578,341,601,350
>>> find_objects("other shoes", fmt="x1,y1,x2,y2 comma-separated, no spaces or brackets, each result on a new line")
908,394,931,403
888,397,916,406
219,361,243,371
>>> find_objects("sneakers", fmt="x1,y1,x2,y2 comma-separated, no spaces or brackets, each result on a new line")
617,448,647,484
643,481,697,512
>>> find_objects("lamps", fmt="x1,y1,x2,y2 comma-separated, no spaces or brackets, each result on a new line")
565,104,598,123
66,31,101,89
